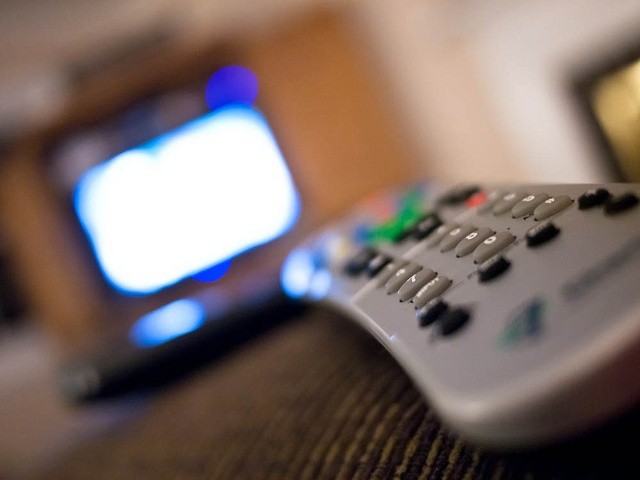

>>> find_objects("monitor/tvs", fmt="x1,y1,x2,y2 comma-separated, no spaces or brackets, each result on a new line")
71,101,300,298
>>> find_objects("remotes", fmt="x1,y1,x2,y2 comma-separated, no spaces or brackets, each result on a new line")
276,175,636,450
